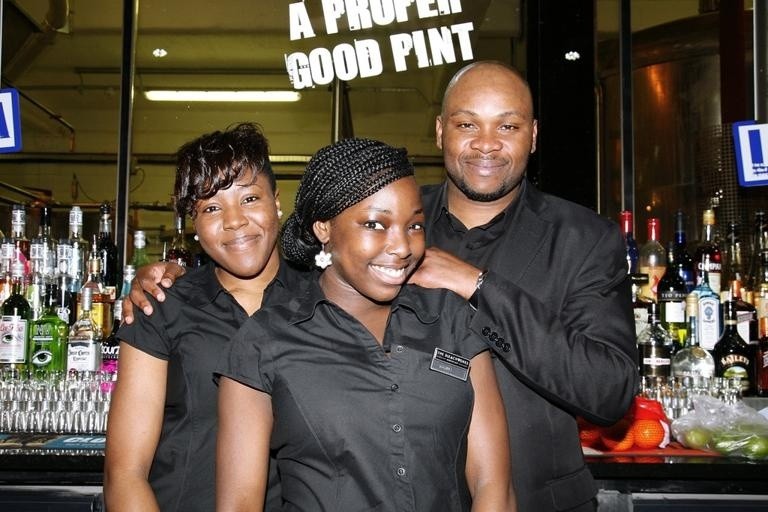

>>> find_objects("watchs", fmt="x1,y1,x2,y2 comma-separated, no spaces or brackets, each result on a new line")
468,267,490,310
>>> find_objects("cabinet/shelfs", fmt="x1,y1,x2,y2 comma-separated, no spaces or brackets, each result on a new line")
584,449,768,510
1,433,106,511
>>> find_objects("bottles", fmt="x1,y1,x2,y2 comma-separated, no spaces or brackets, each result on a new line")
0,366,119,436
0,200,203,382
635,375,742,417
613,204,767,398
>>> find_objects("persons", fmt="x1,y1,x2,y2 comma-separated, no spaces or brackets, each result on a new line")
120,59,641,512
103,122,289,512
213,139,517,512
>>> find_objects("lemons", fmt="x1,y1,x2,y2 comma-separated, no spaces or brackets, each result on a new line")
684,421,768,462
